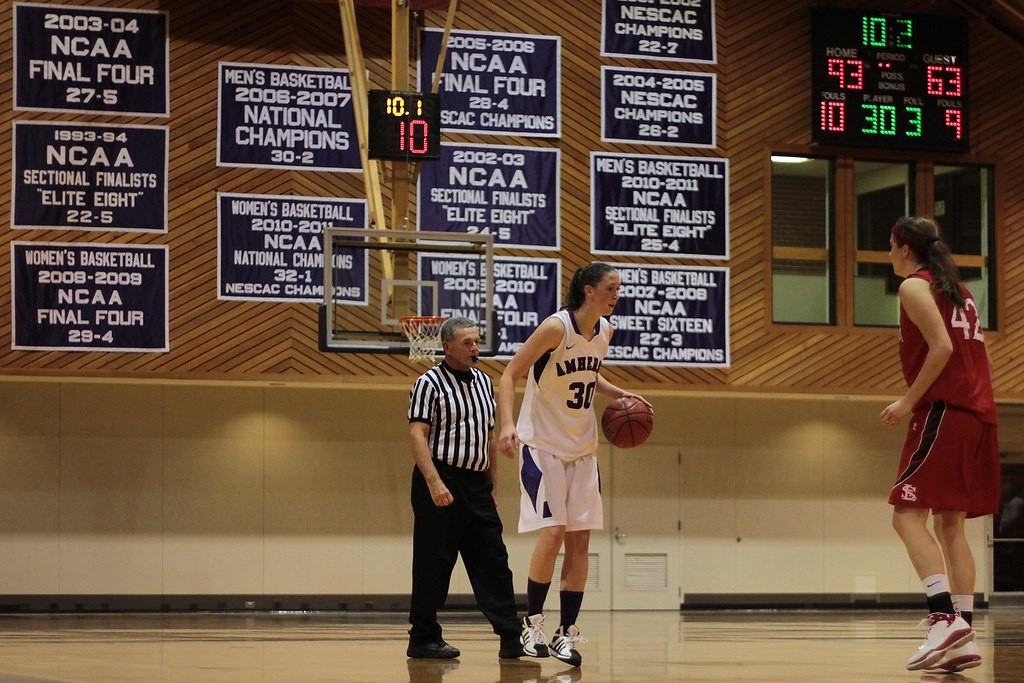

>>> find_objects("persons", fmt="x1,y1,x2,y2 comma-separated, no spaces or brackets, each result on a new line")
873,214,1004,675
497,262,654,669
404,316,543,659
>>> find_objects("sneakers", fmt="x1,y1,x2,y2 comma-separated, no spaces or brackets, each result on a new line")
497,636,551,659
921,638,983,674
549,624,582,667
520,613,549,658
905,612,976,672
406,640,461,659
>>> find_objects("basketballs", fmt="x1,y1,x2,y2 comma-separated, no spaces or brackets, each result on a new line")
601,396,653,448
